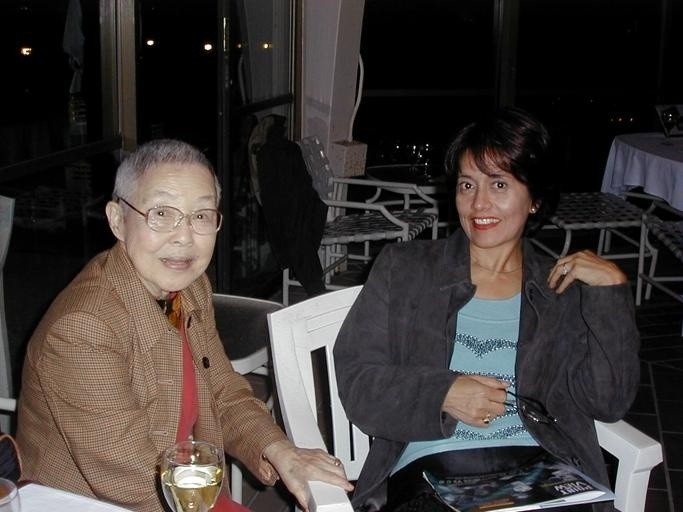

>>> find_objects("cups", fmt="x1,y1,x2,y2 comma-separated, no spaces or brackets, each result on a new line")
159,442,225,512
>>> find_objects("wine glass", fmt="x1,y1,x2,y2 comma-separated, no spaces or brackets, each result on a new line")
661,111,679,145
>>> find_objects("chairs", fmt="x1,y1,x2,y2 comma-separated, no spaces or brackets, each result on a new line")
265,283,664,512
293,135,683,316
210,269,300,511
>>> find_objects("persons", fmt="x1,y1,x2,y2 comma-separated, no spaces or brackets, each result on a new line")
11,137,357,512
328,109,644,512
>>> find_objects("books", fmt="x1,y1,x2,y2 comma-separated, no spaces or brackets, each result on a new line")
421,461,607,512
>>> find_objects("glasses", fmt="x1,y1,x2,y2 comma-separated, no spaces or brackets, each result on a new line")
118,195,224,236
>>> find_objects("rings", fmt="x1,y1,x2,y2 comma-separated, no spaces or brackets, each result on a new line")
483,413,491,424
561,263,568,275
333,458,341,467
